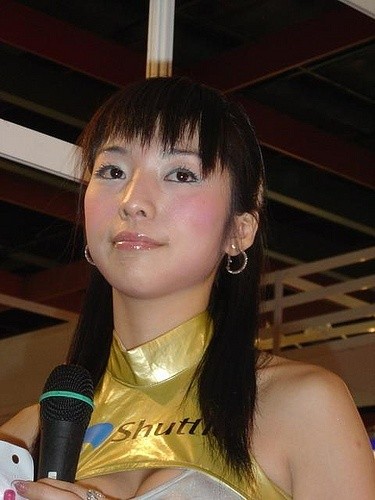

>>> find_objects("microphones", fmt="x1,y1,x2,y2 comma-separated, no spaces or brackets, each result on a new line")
34,362,96,485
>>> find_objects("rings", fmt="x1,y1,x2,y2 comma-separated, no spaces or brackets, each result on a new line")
87,489,105,500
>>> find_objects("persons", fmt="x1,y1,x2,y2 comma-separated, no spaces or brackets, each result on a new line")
0,76,375,500
